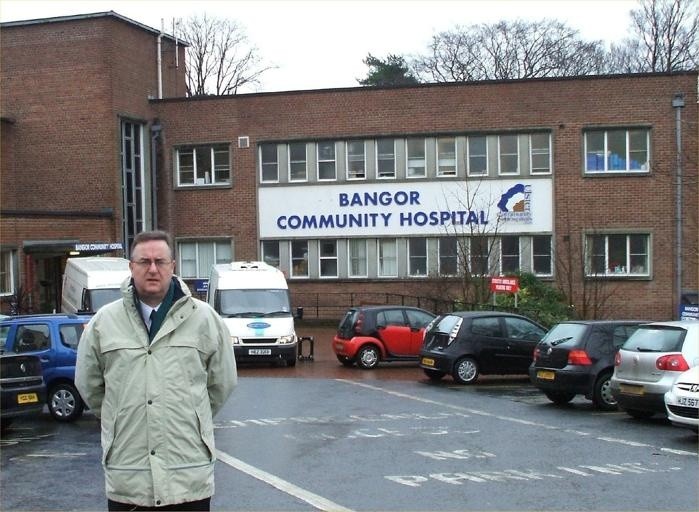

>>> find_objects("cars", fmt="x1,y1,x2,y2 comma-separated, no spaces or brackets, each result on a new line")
0,316,94,421
333,305,437,370
611,319,699,419
0,354,46,427
417,311,550,385
529,320,656,410
664,365,699,435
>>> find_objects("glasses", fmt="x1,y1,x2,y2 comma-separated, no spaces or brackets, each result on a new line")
131,260,172,267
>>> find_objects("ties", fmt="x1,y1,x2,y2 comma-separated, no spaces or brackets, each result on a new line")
150,310,156,333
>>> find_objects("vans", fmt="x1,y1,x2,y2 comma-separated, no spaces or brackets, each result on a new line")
61,256,131,315
206,261,304,366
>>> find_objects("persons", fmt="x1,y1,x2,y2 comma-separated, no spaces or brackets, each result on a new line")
74,231,238,512
